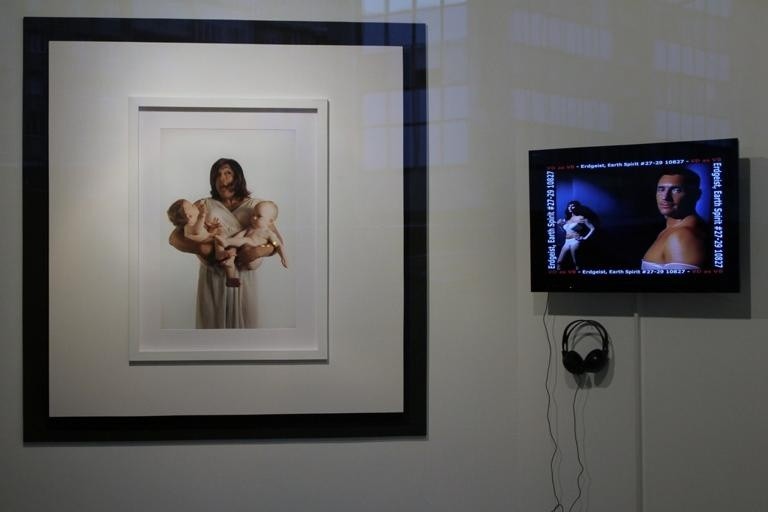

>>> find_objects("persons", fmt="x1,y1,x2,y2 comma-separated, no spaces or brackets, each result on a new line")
555,200,595,272
212,200,288,270
166,198,241,287
640,167,712,272
169,158,283,329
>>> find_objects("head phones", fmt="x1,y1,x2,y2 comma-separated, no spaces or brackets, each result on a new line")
561,319,608,375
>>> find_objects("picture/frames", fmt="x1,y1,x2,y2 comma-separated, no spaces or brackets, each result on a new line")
125,96,330,366
20,16,430,446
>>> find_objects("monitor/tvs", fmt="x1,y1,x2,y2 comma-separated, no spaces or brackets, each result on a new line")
528,137,742,294
